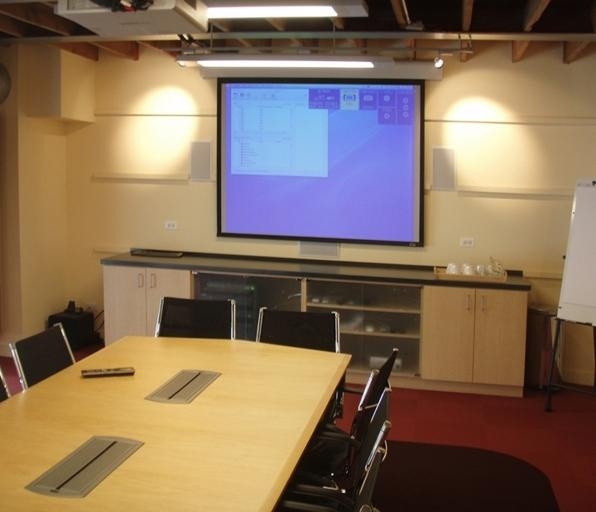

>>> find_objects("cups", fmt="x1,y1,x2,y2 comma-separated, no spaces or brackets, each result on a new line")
445,263,498,275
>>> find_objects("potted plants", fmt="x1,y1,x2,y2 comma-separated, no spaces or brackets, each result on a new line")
50,300,95,349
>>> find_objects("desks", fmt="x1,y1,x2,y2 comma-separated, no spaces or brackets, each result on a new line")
0,337,353,510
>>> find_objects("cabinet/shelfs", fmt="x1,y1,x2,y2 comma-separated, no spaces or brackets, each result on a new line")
101,261,194,347
99,248,531,398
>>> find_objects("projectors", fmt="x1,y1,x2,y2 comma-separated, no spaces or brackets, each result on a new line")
52,1,210,41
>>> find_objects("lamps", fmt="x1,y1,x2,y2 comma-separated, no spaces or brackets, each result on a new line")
433,49,444,70
175,1,396,69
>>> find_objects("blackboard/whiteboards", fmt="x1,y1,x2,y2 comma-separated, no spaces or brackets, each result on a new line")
557,182,596,327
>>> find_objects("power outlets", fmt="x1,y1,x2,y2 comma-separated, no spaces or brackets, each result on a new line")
459,239,473,247
164,222,176,230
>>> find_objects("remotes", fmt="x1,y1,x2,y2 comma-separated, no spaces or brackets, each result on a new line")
80,366,136,378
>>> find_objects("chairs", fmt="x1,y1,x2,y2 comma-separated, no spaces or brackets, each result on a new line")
0,374,11,402
155,296,235,341
282,420,391,510
253,304,345,419
300,373,393,477
10,322,77,388
357,347,397,400
